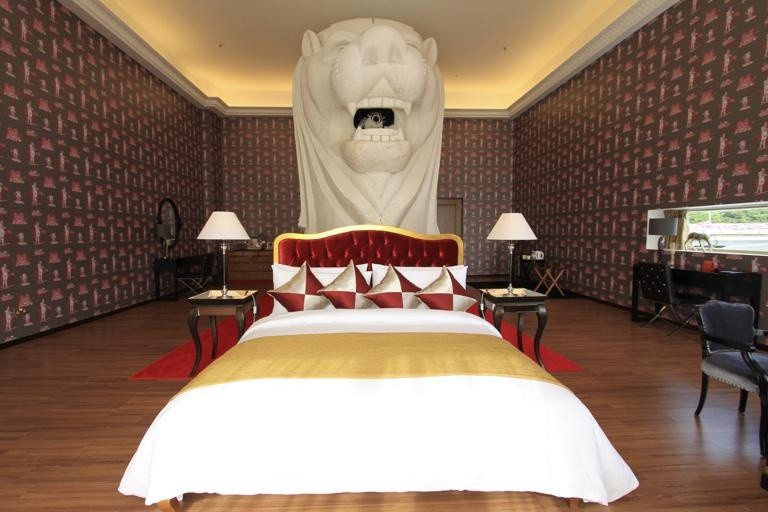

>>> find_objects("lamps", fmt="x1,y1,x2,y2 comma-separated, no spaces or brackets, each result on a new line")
486,213,537,297
649,218,678,249
156,224,172,260
196,211,250,298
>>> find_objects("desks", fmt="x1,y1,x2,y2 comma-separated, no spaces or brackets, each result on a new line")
632,261,761,346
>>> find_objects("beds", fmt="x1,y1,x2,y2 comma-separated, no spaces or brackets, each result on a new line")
119,224,638,510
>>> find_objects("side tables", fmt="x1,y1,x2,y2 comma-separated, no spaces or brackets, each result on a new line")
479,288,549,367
188,290,258,378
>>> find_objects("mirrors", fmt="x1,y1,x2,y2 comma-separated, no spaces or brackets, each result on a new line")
158,199,182,249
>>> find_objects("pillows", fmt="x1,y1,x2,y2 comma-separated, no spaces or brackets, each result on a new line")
271,262,372,312
317,260,373,309
363,264,422,309
372,263,468,290
414,265,477,312
266,260,330,312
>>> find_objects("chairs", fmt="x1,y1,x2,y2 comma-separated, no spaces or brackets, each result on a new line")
636,253,703,336
695,299,768,457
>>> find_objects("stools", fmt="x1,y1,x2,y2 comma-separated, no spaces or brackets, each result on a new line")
176,273,209,297
533,266,564,296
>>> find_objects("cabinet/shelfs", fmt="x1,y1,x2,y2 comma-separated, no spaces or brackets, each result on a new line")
227,249,274,287
